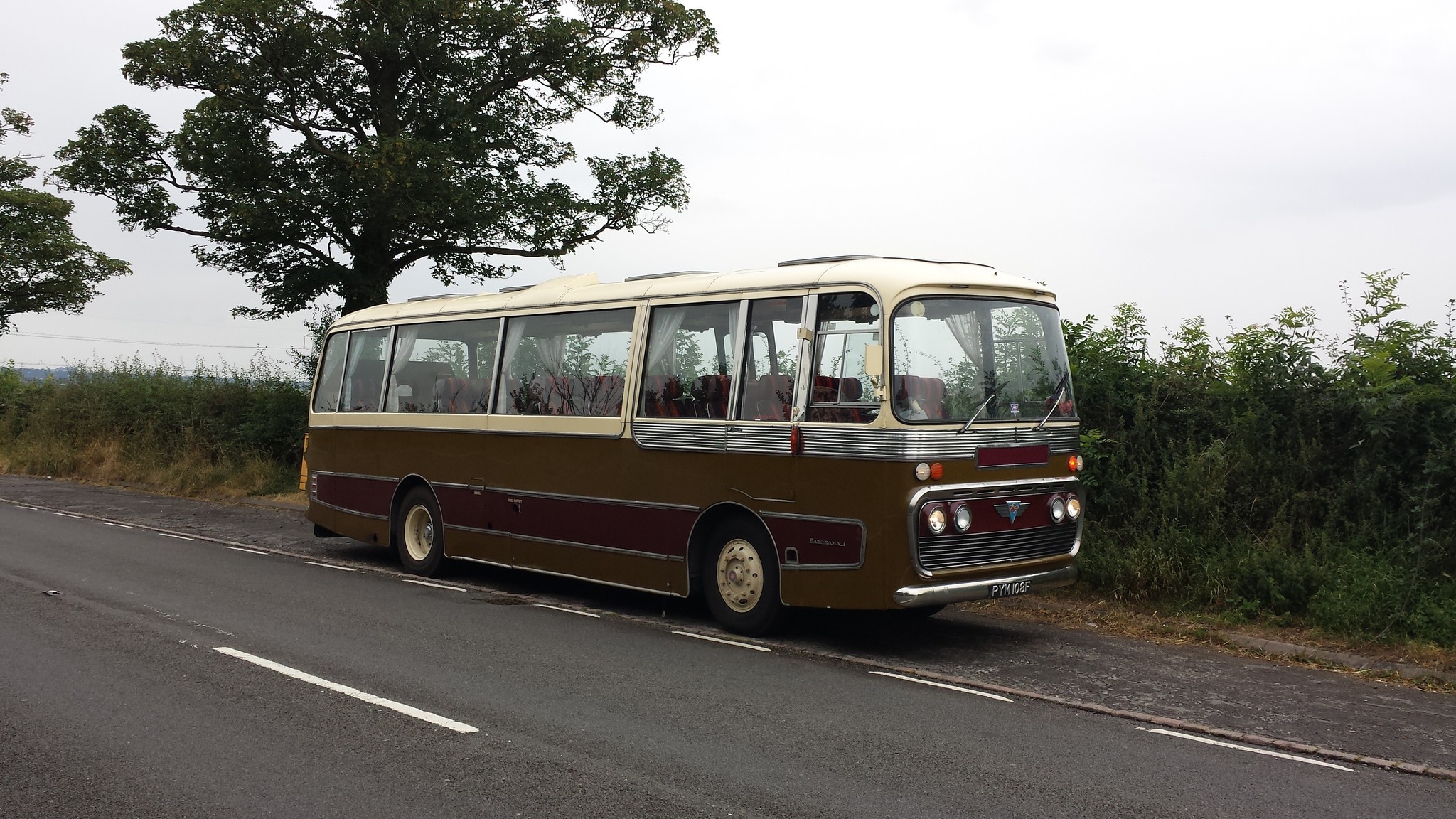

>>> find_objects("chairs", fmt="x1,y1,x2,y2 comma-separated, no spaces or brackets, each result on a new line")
333,370,951,423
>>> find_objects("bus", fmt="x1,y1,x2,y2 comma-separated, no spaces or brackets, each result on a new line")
298,252,1087,638
